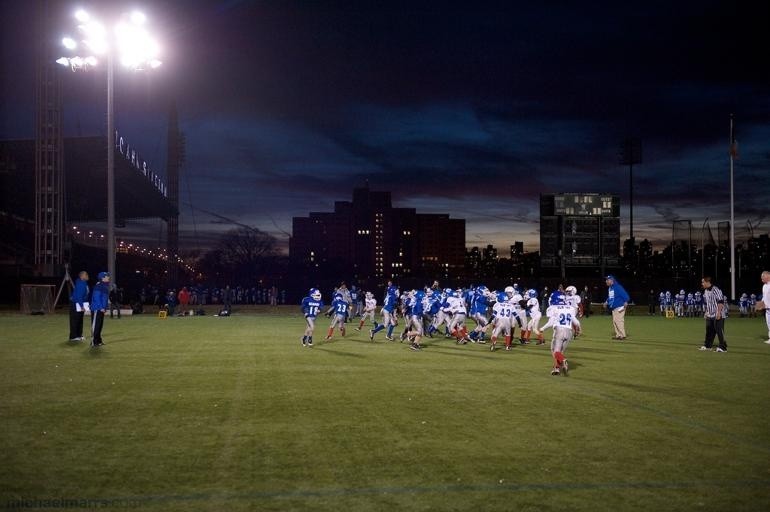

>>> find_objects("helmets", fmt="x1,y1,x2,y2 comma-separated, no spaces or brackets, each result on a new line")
659,289,756,301
309,286,586,304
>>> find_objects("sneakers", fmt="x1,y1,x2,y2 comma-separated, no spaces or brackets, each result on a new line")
70,336,85,341
612,335,626,340
92,342,106,347
697,346,728,352
764,338,770,344
354,327,582,352
302,337,313,346
551,367,560,375
324,335,332,340
647,310,757,318
341,328,346,337
561,359,568,376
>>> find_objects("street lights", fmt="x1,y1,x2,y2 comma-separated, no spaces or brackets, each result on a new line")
53,1,162,316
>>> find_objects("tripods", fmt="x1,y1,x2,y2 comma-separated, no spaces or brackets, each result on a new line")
53,274,76,308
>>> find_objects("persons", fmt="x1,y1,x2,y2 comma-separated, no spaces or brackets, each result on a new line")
139,284,285,309
757,270,770,345
648,289,706,318
700,276,728,352
108,282,122,319
739,293,757,317
540,299,581,375
604,275,630,339
90,271,111,346
301,280,589,356
68,271,89,343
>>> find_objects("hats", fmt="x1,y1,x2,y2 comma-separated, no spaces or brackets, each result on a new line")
96,272,112,280
